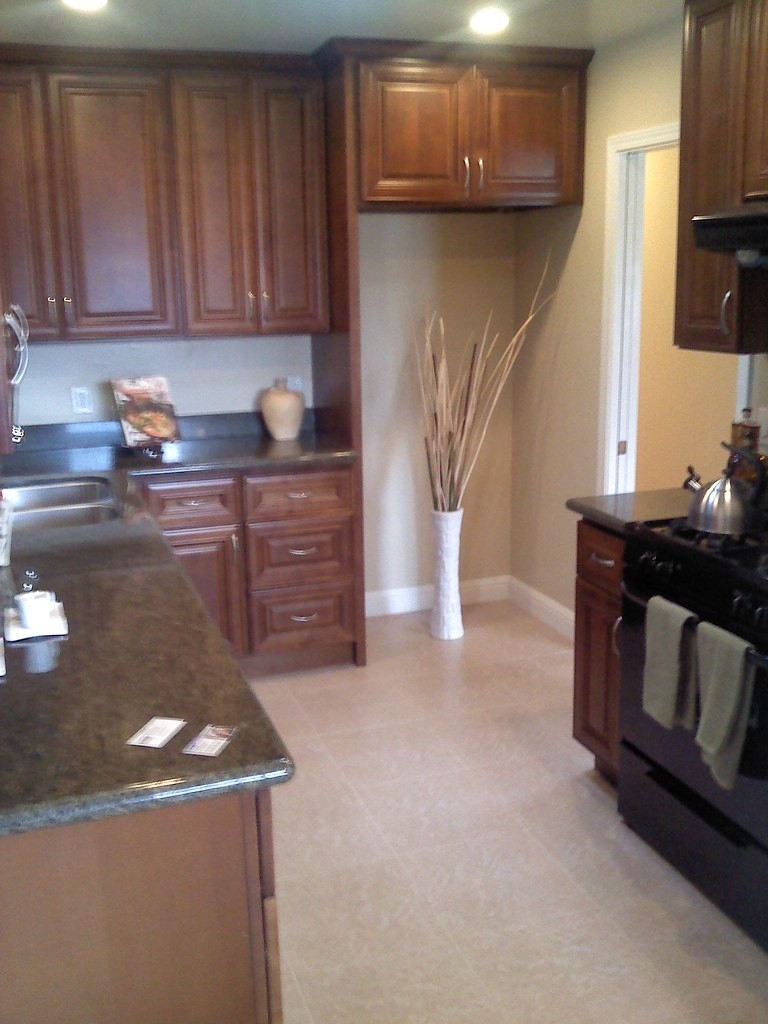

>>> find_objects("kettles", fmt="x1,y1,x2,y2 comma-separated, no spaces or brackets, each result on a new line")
681,440,766,535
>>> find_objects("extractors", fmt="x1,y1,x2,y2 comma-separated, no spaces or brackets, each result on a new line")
692,166,768,264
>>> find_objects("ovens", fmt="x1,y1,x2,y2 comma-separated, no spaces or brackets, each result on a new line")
616,535,768,953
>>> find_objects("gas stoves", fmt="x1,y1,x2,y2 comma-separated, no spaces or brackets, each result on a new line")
639,452,768,597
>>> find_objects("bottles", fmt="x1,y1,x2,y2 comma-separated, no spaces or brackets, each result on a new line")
261,378,305,441
730,406,759,481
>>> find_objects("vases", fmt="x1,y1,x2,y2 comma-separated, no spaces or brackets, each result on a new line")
430,509,465,640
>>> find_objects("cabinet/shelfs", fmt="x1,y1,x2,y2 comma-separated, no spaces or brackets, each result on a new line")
0,784,283,1023
1,258,29,455
169,66,330,334
0,65,178,345
674,0,768,355
354,36,579,212
143,464,368,677
573,520,627,772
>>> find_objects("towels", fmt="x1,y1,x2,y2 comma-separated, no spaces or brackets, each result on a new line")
641,596,699,732
696,621,755,795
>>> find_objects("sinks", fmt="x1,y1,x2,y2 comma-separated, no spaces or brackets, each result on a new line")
1,474,114,509
